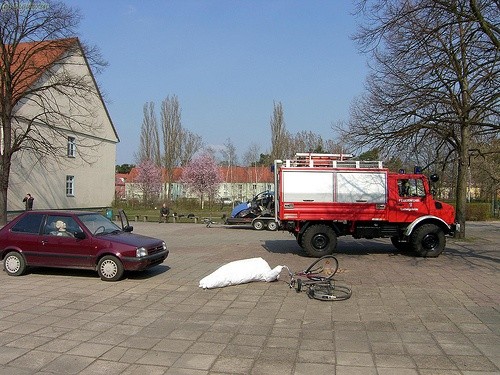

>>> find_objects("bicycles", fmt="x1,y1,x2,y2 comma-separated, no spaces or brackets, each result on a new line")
282,255,352,300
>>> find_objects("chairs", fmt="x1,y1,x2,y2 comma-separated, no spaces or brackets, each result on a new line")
49,221,59,236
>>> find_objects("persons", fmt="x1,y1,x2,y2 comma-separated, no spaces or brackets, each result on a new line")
22,194,34,210
56,218,78,238
159,203,170,223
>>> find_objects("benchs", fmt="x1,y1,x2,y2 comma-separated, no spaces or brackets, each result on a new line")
201,216,221,224
177,216,199,223
115,214,140,222
160,215,177,223
142,214,160,223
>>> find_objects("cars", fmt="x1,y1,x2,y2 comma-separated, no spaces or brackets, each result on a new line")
0,209,170,281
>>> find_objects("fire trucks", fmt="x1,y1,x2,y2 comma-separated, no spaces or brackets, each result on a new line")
274,153,460,258
